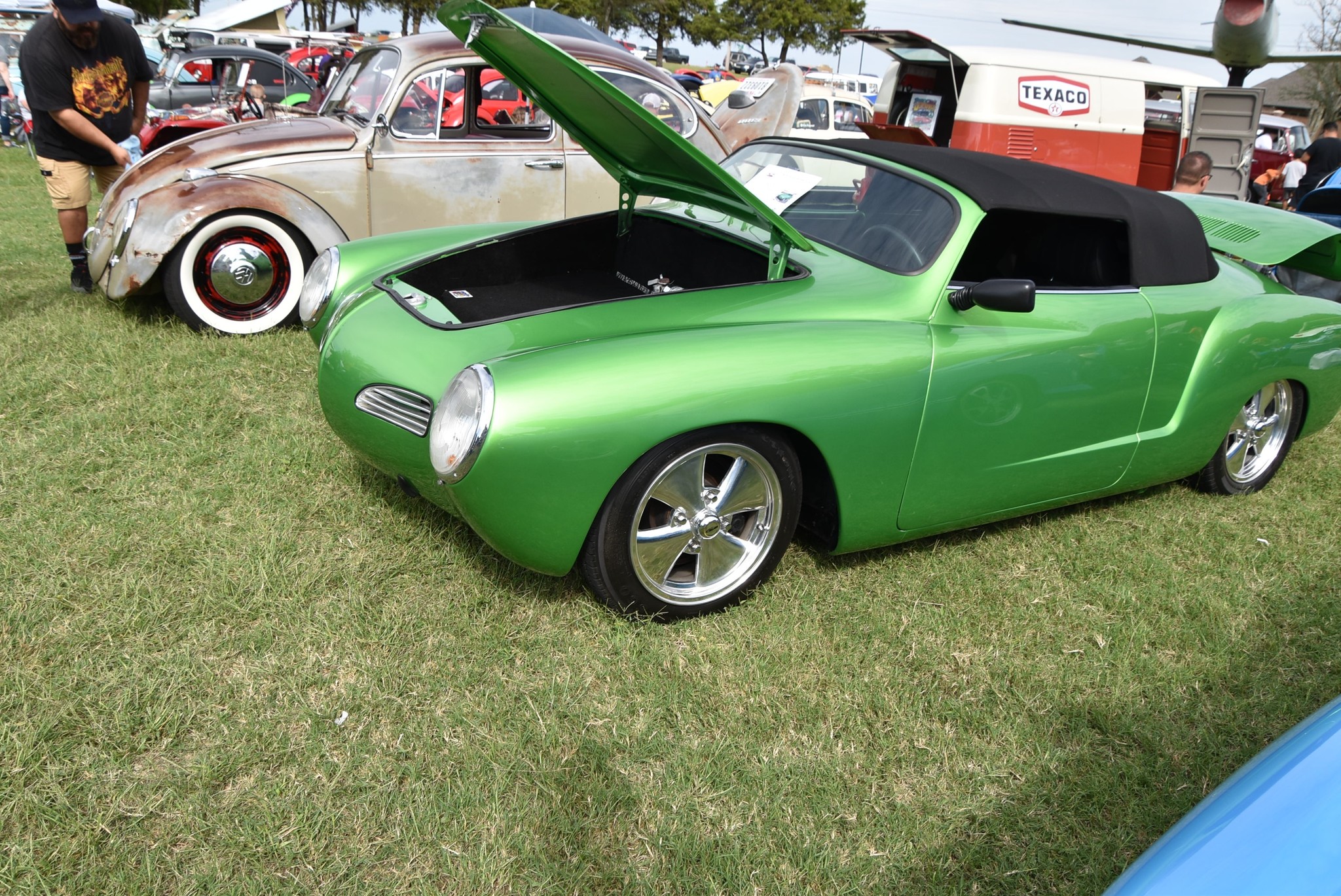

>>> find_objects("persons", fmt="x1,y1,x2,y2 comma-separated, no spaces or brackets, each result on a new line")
641,92,662,117
0,44,16,146
511,106,526,125
833,102,853,131
1169,150,1213,193
19,0,153,295
1254,128,1279,151
248,83,266,118
1278,148,1307,211
708,63,724,83
317,44,339,72
1288,122,1341,212
1252,164,1286,205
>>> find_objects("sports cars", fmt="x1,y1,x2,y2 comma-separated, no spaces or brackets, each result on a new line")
299,0,1340,626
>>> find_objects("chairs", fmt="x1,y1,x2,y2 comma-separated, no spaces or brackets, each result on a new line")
921,208,1043,283
1010,215,1129,287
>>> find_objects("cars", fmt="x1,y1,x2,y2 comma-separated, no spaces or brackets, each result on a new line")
83,32,750,338
1,0,880,153
1101,697,1341,896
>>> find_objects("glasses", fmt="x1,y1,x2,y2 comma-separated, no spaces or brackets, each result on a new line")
1201,174,1213,181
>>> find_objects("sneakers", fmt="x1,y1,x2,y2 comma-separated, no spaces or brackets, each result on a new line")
71,269,93,293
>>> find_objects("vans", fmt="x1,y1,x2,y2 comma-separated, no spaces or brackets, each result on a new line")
839,29,1268,201
1248,114,1314,204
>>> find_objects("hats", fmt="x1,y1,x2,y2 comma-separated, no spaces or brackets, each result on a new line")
53,0,104,25
